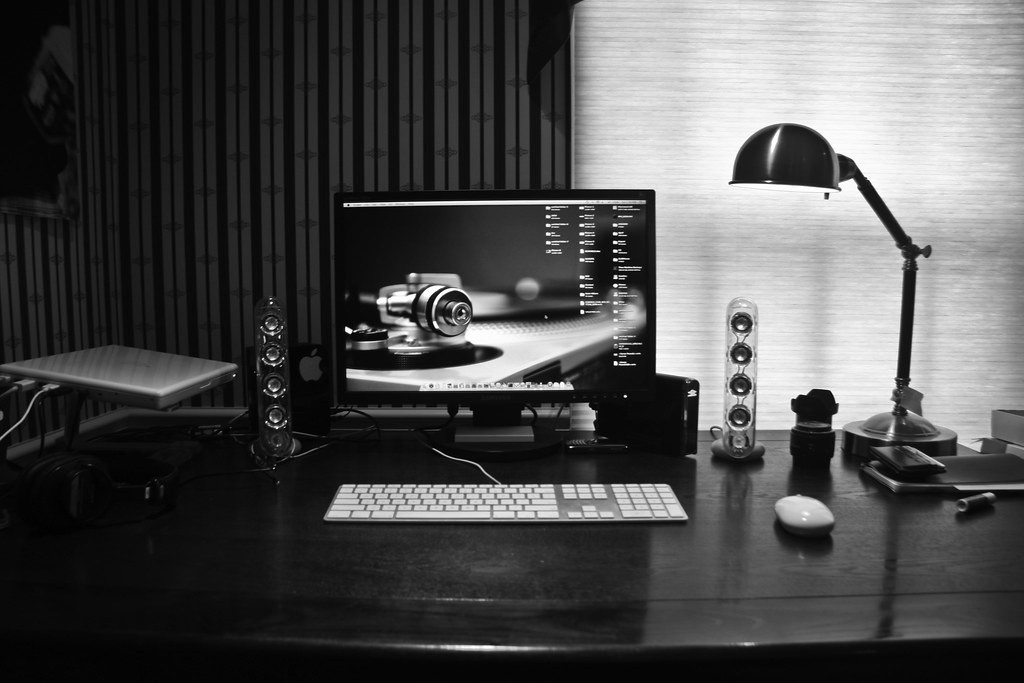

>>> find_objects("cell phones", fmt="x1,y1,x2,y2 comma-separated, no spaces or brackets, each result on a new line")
565,439,631,454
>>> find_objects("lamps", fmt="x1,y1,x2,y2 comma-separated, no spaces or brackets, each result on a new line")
729,123,961,461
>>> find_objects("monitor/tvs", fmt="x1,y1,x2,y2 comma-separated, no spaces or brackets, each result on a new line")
318,189,657,463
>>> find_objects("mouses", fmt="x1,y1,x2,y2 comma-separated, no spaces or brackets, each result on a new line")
774,494,835,537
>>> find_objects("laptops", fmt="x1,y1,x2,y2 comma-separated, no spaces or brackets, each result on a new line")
0,343,237,410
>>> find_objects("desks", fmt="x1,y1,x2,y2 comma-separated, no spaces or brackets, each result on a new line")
0,418,1024,683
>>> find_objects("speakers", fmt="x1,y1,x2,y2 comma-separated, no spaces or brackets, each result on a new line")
711,297,764,462
246,297,304,465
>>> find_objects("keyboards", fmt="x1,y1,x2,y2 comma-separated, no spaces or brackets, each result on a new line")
324,483,687,521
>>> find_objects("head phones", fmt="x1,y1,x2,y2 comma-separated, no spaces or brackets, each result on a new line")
11,449,180,533
